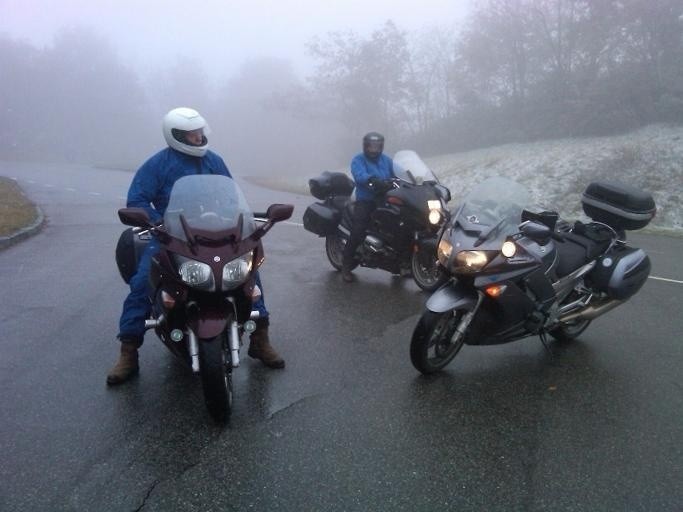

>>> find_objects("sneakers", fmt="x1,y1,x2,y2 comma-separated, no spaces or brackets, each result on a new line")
342,266,353,284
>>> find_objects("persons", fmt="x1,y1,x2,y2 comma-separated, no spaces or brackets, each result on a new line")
98,108,288,386
339,132,421,283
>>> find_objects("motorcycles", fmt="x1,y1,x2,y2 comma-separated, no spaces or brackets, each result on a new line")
301,151,453,294
114,174,295,423
409,175,655,375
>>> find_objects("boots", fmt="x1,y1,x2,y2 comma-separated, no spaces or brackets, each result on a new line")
248,326,285,370
106,341,140,387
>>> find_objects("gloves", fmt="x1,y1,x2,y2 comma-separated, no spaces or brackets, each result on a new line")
369,176,387,191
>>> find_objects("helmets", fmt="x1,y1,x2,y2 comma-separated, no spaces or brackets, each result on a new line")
162,107,209,158
363,132,384,160
